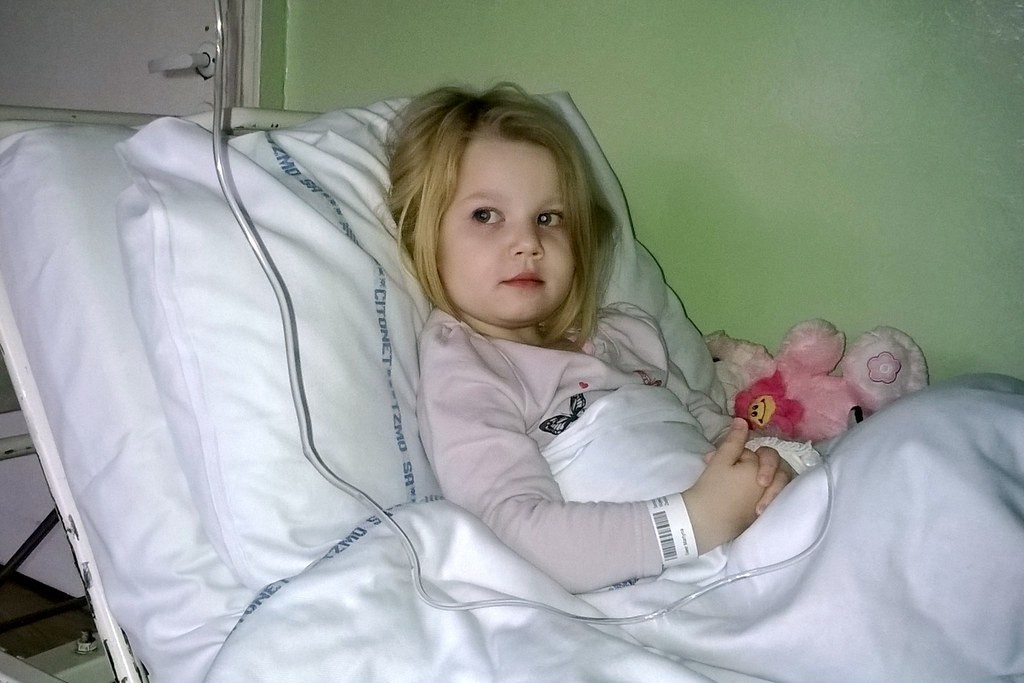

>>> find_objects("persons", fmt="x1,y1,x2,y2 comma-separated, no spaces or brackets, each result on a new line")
385,81,802,597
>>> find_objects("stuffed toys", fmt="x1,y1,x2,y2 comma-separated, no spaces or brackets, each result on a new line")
701,318,929,440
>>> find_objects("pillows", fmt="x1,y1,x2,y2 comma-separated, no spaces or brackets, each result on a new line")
116,92,718,601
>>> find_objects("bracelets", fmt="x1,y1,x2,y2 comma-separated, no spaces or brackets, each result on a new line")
645,491,698,570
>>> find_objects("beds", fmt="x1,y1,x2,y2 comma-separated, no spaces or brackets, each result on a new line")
1,90,1024,681
